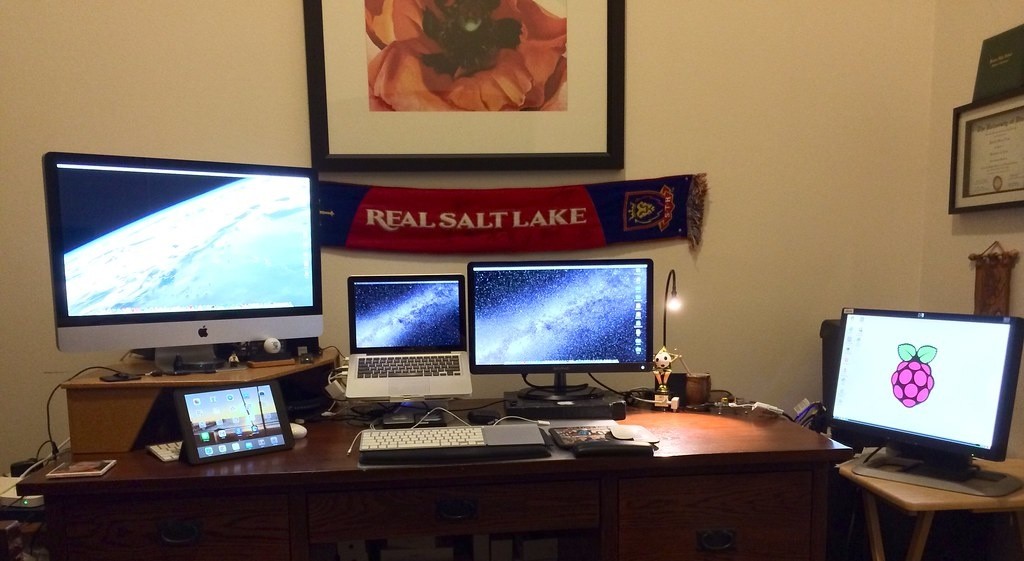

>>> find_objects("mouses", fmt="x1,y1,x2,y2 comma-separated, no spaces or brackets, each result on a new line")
609,426,634,439
290,423,307,439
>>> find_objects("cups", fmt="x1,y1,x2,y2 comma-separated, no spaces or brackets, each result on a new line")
687,373,711,405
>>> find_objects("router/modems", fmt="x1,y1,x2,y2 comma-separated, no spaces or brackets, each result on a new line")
0,477,44,508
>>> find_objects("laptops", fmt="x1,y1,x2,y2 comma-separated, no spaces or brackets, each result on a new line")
345,273,472,402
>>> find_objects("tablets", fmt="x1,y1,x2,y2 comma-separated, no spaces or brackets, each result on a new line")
174,380,294,465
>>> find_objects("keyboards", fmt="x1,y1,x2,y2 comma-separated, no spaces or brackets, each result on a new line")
359,426,486,450
146,441,183,462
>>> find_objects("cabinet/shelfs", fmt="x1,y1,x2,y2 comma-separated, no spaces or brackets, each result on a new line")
16,398,853,561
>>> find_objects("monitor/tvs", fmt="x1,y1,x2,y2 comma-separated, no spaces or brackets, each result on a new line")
827,308,1024,496
42,151,323,376
467,258,653,401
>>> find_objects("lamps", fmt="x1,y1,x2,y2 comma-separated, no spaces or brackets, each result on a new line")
662,269,676,348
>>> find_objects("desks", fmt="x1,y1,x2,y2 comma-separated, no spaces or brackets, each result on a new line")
839,453,1024,561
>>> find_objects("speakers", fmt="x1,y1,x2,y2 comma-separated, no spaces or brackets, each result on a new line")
820,318,864,453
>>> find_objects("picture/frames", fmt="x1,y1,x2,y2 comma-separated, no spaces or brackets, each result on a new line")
948,86,1024,214
303,0,626,170
